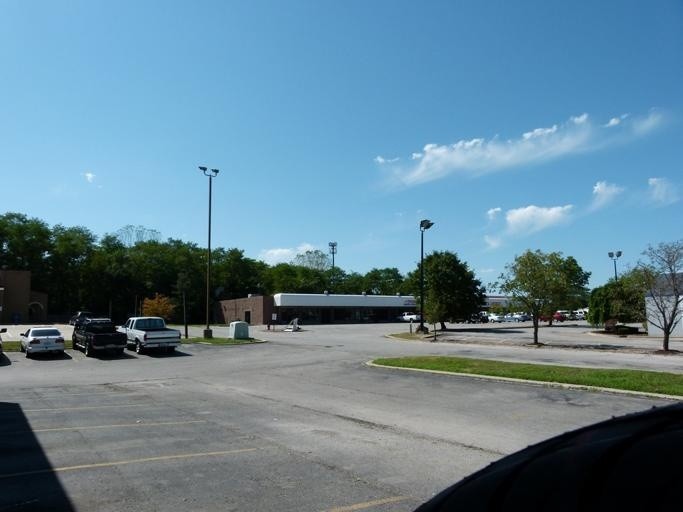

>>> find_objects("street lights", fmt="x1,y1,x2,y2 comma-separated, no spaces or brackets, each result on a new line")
328,240,336,292
197,164,219,338
607,251,622,281
415,219,434,335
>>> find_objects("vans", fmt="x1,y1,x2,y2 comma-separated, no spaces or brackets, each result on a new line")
69,310,93,326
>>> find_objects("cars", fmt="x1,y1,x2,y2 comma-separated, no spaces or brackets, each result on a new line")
19,327,65,360
472,310,589,324
82,318,111,324
0,327,7,353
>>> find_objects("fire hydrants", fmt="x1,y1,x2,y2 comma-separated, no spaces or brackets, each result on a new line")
267,323,270,330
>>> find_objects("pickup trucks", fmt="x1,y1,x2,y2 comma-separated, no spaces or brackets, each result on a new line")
71,320,127,357
395,312,420,322
114,317,181,353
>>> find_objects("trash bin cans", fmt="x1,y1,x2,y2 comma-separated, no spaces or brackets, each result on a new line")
12,313,20,325
230,320,249,339
